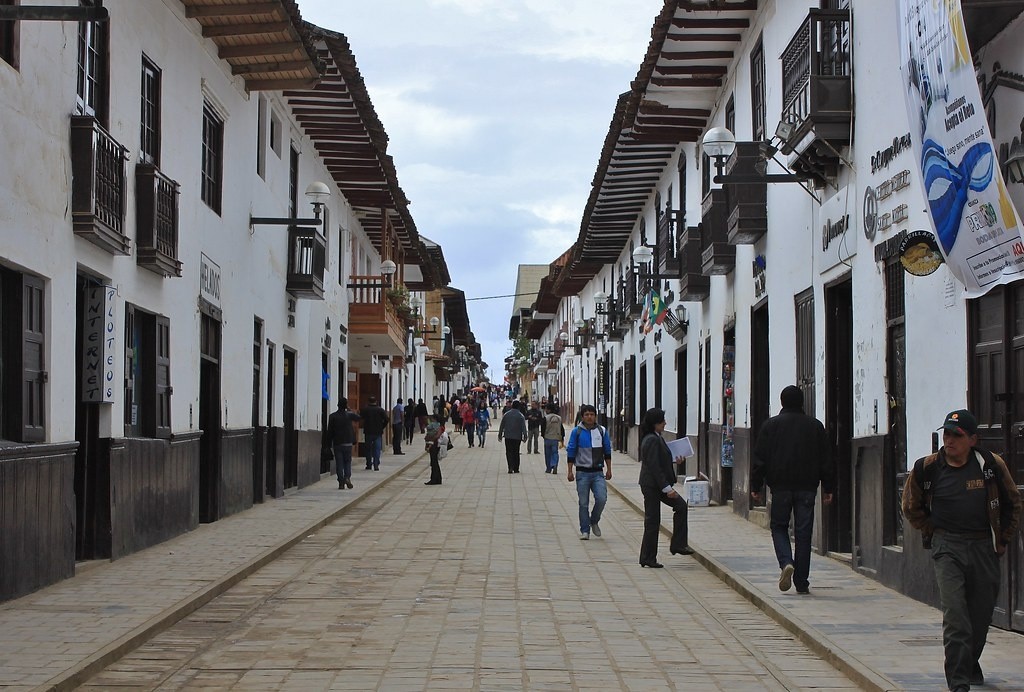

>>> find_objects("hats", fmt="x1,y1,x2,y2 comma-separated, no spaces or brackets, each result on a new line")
937,408,977,436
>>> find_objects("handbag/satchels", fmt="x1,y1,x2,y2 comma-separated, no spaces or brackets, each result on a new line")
447,437,453,450
443,407,449,417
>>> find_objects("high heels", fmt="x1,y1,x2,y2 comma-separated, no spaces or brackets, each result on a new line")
641,560,665,569
670,545,694,556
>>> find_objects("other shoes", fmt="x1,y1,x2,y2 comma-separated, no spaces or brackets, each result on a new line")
779,565,794,592
365,467,371,469
534,450,539,454
545,469,552,473
482,445,484,448
395,453,406,455
425,480,442,485
591,520,601,537
553,467,557,474
471,442,474,447
969,660,985,685
581,531,589,540
797,588,808,593
344,479,354,489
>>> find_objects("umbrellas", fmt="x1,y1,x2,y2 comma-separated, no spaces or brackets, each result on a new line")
471,387,484,391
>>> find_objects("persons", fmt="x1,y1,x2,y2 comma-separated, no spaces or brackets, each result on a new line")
574,404,585,426
567,405,612,540
750,385,835,594
638,409,694,567
328,398,361,489
902,409,1024,692
541,403,565,474
391,398,405,455
404,398,418,444
433,382,545,448
361,396,388,470
417,399,428,434
525,401,542,454
423,414,445,485
498,401,527,473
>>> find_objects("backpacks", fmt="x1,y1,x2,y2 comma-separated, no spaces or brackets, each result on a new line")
450,405,458,417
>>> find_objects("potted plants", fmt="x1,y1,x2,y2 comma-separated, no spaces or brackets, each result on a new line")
387,281,425,337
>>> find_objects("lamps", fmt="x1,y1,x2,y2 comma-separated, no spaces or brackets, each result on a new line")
702,126,807,182
560,331,580,347
250,182,330,224
675,308,689,325
574,318,606,336
347,259,396,288
427,326,451,340
410,297,423,315
594,291,623,315
632,246,680,280
409,317,439,333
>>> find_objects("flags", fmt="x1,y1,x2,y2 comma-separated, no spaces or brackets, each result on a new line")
642,289,667,335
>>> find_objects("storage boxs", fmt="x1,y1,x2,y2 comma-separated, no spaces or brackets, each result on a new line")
683,472,712,506
